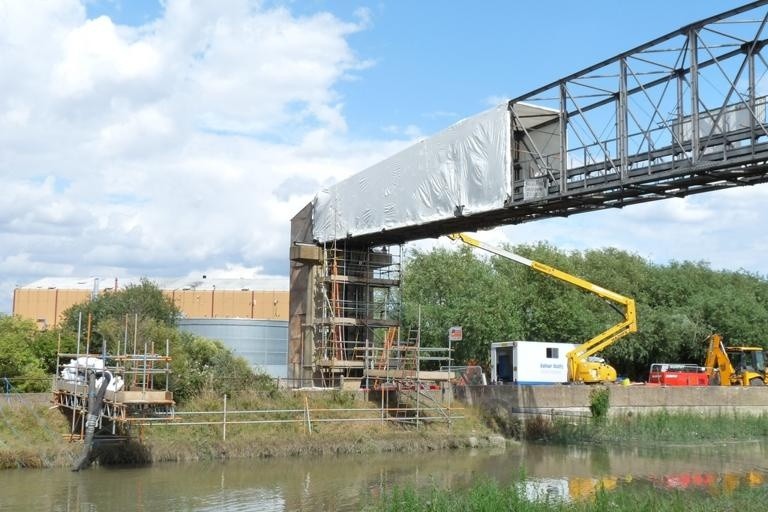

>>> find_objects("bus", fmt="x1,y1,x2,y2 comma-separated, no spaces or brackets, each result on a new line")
649,363,721,386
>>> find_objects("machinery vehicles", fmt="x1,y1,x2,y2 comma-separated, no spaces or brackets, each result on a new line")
702,333,768,386
449,233,639,383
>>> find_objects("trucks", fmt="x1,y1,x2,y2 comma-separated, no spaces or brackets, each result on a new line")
491,340,606,384
